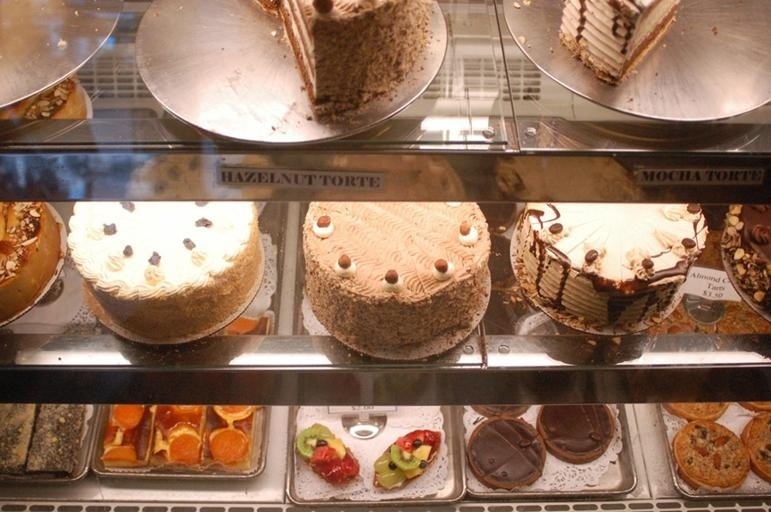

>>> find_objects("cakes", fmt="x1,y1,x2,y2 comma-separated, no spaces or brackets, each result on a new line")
0,76,86,120
0,202,61,322
465,403,616,492
373,430,442,493
514,202,709,324
296,422,361,487
251,0,432,125
722,203,771,314
558,0,682,87
302,201,492,347
66,200,261,339
662,401,771,492
0,401,256,482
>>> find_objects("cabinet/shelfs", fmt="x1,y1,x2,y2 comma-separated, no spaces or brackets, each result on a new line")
0,0,771,512
